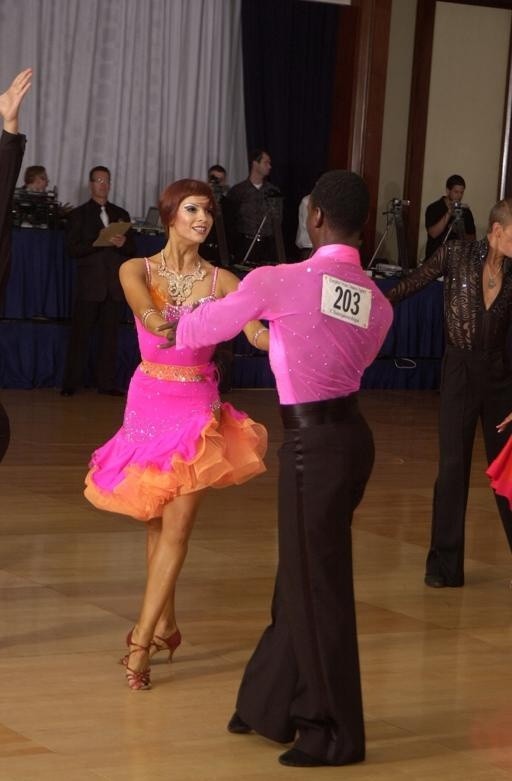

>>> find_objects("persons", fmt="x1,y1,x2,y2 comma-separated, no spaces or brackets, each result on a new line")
0,68,32,462
426,175,476,260
61,166,137,397
156,171,394,767
295,195,314,259
224,151,282,261
199,165,228,258
82,179,269,690
15,165,50,203
484,413,512,509
383,199,512,588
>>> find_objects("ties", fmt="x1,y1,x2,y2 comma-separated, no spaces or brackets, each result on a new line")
99,205,110,229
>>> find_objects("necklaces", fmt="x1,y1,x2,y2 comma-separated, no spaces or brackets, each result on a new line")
486,257,503,289
157,250,206,306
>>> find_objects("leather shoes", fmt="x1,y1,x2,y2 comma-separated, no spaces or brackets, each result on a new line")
278,750,333,767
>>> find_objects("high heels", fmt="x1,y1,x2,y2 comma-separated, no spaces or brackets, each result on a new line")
116,622,181,691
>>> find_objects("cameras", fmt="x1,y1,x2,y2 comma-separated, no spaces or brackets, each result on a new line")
392,198,411,208
453,200,469,209
209,174,222,185
